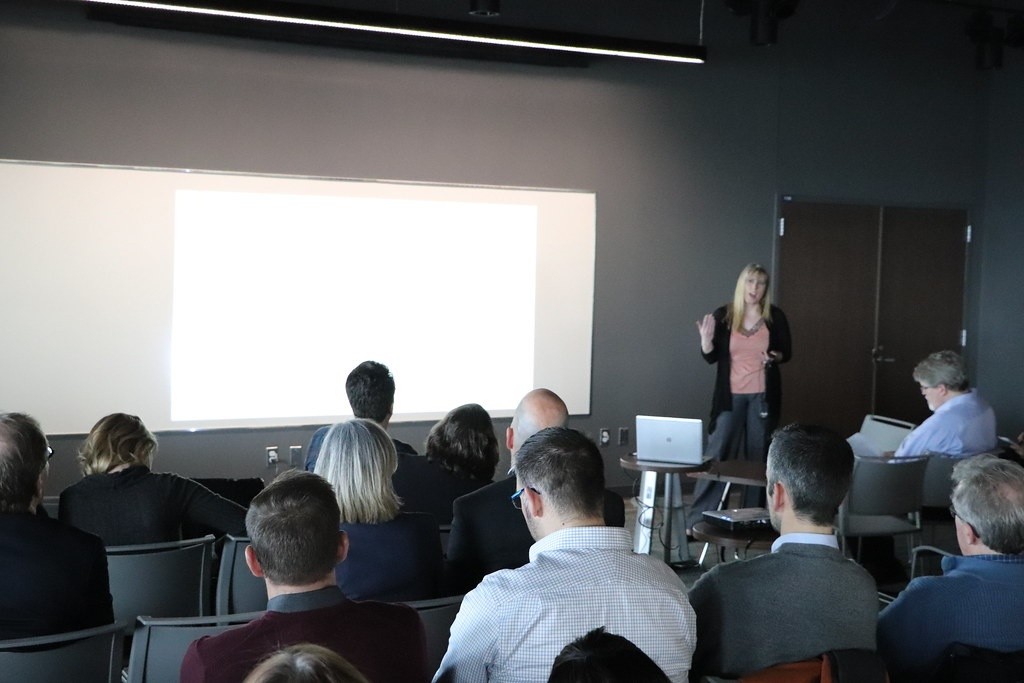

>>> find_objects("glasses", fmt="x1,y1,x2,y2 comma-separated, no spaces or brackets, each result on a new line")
511,488,540,509
42,446,55,468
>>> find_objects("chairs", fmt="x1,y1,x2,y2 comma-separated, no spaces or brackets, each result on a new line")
0,619,128,683
830,451,933,606
893,448,1005,578
394,594,464,683
860,414,920,529
103,534,215,669
121,609,268,683
216,533,269,628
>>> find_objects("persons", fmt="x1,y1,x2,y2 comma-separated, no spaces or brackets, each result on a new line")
864,351,997,583
686,263,792,543
391,404,500,526
547,626,673,683
304,361,418,473
0,412,115,640
877,454,1024,683
440,388,625,598
58,413,248,547
431,426,697,683
688,422,879,683
180,468,428,683
243,644,368,683
313,419,443,603
998,430,1024,468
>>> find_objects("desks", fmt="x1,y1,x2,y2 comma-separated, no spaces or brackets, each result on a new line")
619,449,715,556
690,522,779,551
685,459,766,567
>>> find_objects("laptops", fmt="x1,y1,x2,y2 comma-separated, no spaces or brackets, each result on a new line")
636,415,713,465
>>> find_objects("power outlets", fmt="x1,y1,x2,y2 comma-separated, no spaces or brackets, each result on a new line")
600,427,610,448
618,428,628,447
289,445,302,467
265,446,280,470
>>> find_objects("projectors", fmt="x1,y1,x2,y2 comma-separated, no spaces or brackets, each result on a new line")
701,508,772,531
